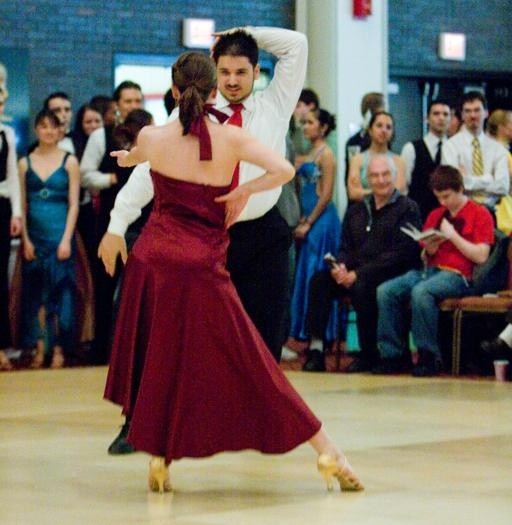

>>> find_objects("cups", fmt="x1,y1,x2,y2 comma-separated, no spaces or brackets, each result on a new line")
492,360,509,381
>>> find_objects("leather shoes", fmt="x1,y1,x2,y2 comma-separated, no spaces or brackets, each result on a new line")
301,347,327,373
108,413,142,456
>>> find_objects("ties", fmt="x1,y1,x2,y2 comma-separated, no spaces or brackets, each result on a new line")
226,104,243,191
471,138,487,205
434,141,442,165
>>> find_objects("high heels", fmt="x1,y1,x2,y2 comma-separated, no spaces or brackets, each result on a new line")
315,451,365,493
148,456,174,496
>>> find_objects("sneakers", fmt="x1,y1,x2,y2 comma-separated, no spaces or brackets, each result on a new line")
1,347,109,375
342,349,442,378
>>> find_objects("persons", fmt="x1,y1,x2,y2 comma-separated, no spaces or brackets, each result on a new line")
97,26,309,455
111,51,363,496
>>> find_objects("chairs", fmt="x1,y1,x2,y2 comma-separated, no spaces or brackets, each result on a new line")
408,230,505,375
455,237,512,377
